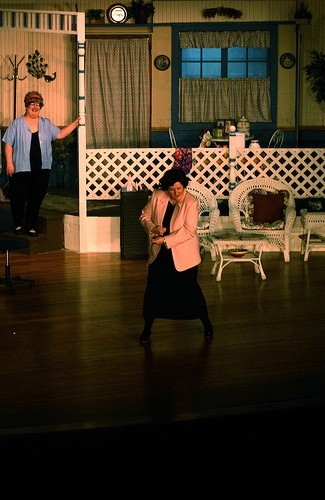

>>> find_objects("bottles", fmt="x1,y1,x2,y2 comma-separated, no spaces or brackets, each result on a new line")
122,173,145,192
213,118,231,138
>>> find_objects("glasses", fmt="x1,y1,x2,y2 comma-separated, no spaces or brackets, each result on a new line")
30,102,41,107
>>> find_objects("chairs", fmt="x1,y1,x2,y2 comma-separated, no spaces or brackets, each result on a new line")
185,182,220,262
168,127,178,148
229,179,296,262
268,129,285,149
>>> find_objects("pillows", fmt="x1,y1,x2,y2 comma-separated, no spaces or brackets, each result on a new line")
252,193,285,223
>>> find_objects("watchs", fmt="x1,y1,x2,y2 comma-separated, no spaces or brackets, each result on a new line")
164,240,167,245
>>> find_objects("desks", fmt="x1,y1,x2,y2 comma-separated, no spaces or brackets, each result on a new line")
299,211,325,262
200,131,254,141
207,233,267,281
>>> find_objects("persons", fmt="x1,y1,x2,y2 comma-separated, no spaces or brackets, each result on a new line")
138,167,214,345
2,91,81,237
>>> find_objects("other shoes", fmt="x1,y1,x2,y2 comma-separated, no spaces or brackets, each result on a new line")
15,226,23,235
28,229,39,237
204,325,214,339
140,329,151,343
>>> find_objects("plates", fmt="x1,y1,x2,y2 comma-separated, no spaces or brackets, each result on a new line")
229,250,248,258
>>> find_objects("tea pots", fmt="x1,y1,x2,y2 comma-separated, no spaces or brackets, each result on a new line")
237,115,250,136
